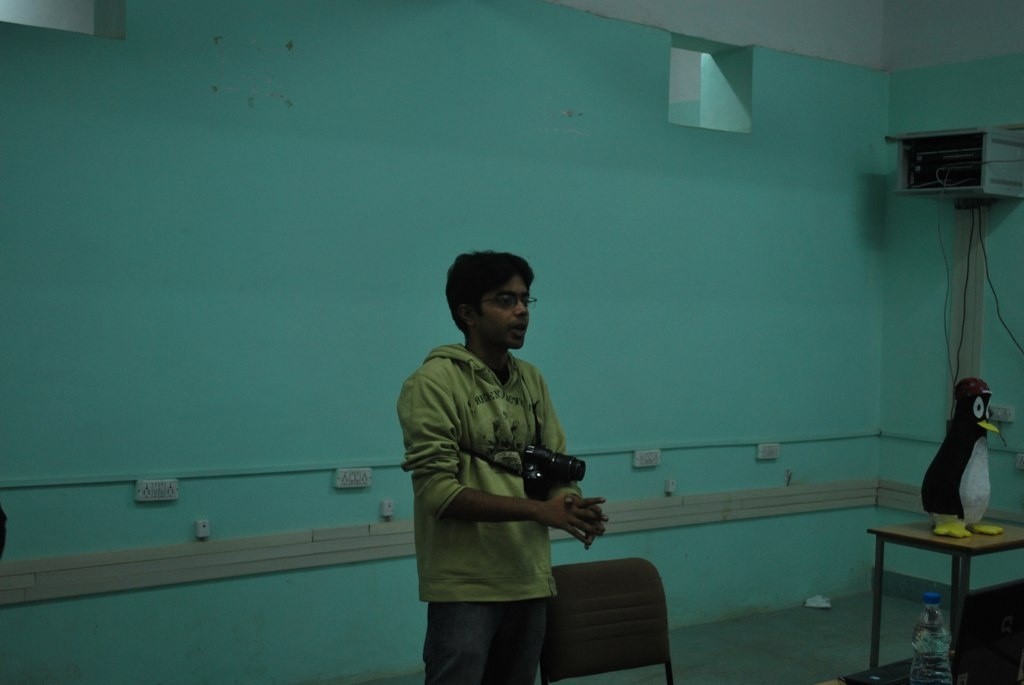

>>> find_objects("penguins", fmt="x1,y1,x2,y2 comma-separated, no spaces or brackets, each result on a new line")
921,393,1005,537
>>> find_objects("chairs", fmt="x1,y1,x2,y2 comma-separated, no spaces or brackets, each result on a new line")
538,558,674,685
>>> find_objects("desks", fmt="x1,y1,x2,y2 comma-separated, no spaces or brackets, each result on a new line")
866,520,1024,671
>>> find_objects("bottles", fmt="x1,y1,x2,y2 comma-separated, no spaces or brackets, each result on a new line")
910,590,952,685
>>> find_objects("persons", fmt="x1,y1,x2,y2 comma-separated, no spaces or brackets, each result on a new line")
396,250,608,685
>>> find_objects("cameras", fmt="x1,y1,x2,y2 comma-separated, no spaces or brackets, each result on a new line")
522,443,585,499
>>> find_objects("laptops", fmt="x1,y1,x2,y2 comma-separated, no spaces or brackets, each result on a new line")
844,576,1024,685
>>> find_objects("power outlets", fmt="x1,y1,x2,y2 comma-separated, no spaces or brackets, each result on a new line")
134,477,178,500
634,450,660,467
756,443,779,459
335,467,371,488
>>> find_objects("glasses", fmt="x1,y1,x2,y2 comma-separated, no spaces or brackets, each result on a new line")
470,292,538,312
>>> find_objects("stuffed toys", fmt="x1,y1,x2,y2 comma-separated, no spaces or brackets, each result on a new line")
921,377,1004,538
